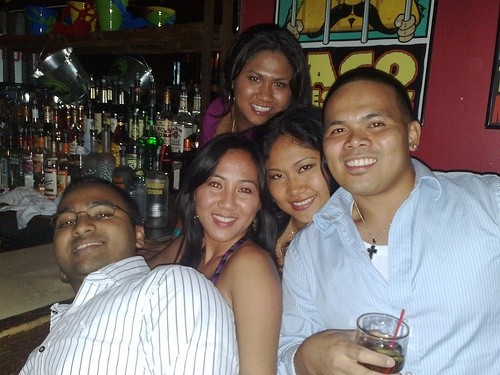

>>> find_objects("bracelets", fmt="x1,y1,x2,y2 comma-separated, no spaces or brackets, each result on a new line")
174,228,181,238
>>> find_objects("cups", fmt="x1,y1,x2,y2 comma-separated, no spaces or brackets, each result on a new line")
356,313,410,374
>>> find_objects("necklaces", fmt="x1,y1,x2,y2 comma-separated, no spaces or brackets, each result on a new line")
350,186,414,260
289,222,298,239
230,115,237,135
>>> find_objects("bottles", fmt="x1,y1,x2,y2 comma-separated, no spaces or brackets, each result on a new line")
112,150,169,228
44,141,72,201
44,105,63,155
189,88,202,149
63,104,84,155
169,90,193,154
0,96,22,194
155,88,174,152
19,132,45,193
20,100,44,151
89,77,159,138
112,114,164,169
73,112,116,184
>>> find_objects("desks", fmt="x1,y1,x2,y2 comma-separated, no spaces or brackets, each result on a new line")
0,232,164,375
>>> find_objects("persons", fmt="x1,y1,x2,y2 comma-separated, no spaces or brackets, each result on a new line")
16,174,241,375
172,21,313,242
272,59,500,375
252,104,343,288
137,131,282,375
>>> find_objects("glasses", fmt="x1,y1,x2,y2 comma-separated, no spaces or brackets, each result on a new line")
47,202,141,230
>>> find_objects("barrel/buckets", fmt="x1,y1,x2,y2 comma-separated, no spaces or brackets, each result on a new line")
4,13,25,34
96,0,128,32
25,7,58,34
144,7,176,26
101,52,154,112
30,40,93,104
68,1,97,32
